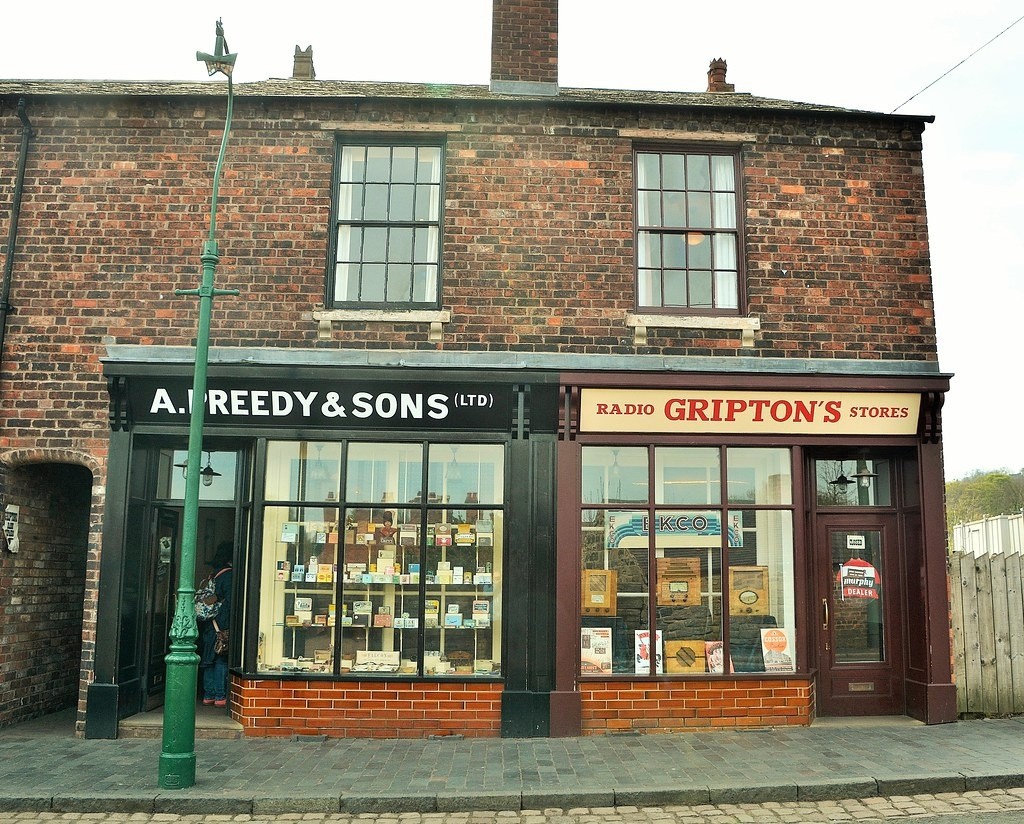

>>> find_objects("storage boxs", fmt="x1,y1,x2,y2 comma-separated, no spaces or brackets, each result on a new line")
276,520,493,672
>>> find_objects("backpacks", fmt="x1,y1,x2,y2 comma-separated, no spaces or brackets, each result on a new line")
193,566,233,621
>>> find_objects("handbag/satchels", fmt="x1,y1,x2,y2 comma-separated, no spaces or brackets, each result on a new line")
214,629,230,657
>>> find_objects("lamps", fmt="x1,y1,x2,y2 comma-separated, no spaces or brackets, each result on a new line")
310,445,326,479
445,446,461,479
173,444,204,480
610,449,622,476
200,451,222,486
829,460,856,494
850,446,879,488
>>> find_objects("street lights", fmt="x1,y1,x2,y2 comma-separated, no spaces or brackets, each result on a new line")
159,15,241,790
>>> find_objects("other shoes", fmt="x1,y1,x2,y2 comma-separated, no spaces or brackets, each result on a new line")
202,699,214,706
214,698,227,707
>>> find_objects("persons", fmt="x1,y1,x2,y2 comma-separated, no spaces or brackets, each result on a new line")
203,536,234,708
